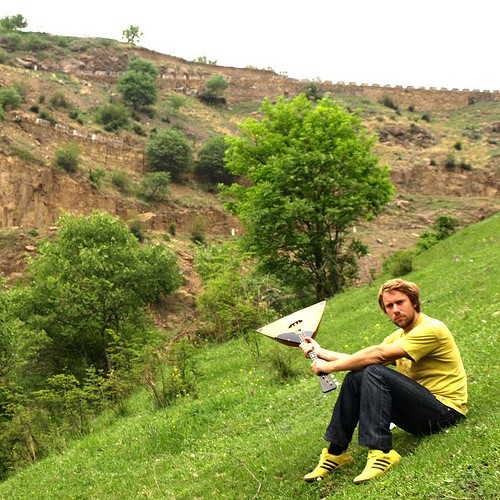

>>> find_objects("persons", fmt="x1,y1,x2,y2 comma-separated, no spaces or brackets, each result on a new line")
298,277,469,484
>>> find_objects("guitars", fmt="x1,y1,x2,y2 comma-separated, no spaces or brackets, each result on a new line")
257,301,339,394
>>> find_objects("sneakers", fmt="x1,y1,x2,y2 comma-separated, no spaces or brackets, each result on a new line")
304,447,353,482
354,450,403,484
